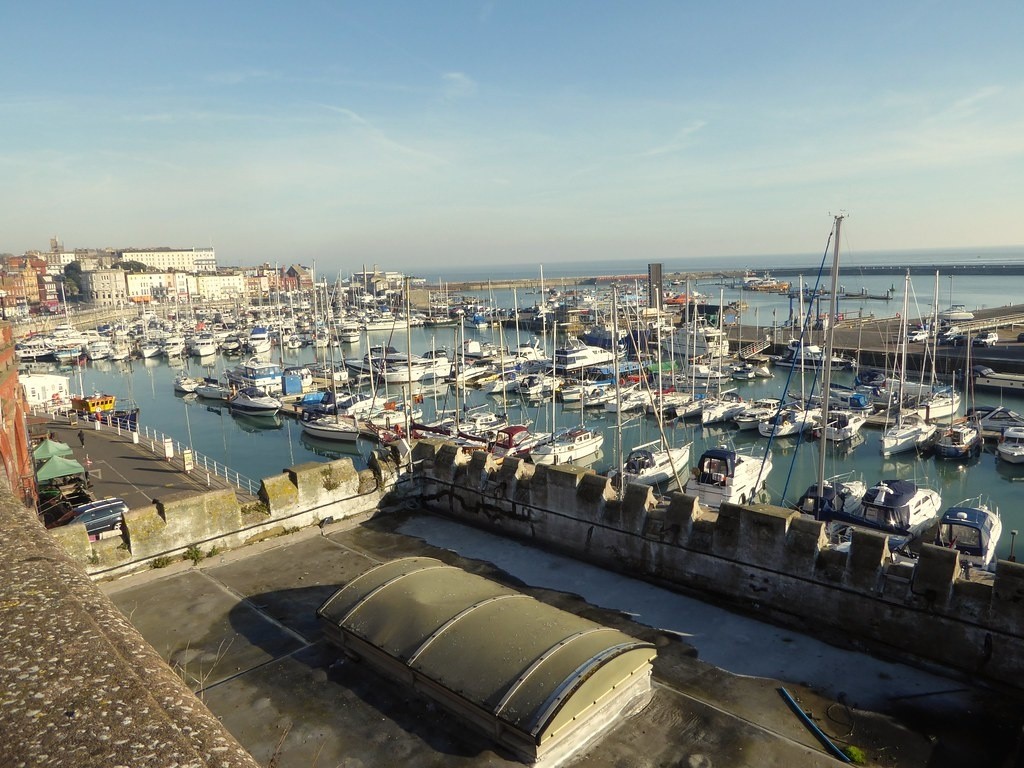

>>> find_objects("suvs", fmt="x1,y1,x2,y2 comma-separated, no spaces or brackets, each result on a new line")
973,331,999,348
45,497,129,537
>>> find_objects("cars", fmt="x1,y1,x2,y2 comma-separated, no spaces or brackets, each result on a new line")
949,335,973,347
940,333,958,346
907,330,927,343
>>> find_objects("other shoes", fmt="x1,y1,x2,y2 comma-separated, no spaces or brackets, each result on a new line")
83,446,84,448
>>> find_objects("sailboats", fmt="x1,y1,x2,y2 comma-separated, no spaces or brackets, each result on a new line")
13,209,1024,572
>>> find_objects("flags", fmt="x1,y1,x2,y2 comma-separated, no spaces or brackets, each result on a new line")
413,394,424,404
383,402,396,410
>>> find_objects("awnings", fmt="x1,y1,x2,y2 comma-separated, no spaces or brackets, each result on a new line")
36,455,85,482
33,439,73,459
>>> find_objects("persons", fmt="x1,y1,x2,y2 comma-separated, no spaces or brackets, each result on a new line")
78,430,85,449
